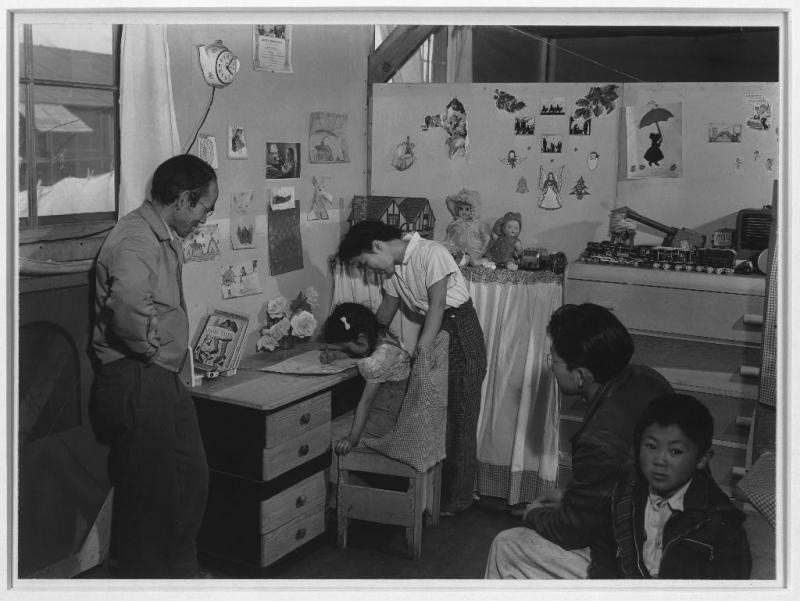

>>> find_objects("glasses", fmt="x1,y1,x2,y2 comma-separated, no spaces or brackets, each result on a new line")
546,352,568,371
178,190,214,218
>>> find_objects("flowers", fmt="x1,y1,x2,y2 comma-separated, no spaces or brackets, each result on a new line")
257,285,321,351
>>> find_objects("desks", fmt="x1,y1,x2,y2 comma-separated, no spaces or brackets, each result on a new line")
330,258,563,505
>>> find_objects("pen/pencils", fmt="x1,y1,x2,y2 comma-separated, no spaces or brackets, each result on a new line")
324,345,329,357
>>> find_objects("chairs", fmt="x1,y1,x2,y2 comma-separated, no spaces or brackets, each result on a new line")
337,330,450,560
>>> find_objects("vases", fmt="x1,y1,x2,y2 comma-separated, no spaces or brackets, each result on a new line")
278,331,295,349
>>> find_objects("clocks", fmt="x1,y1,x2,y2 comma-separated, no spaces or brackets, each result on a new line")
198,39,241,89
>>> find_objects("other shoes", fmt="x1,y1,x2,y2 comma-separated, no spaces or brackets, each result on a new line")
439,494,474,515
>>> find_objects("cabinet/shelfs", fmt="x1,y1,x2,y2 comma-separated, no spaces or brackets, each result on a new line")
557,261,767,514
191,353,368,568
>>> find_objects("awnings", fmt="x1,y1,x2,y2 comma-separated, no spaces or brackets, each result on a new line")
19,103,94,133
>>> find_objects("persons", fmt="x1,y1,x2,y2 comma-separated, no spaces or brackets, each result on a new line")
444,188,488,267
319,302,412,514
485,303,677,579
89,153,218,579
339,217,488,517
232,124,244,152
196,318,238,366
586,393,753,579
486,212,522,272
280,147,295,178
